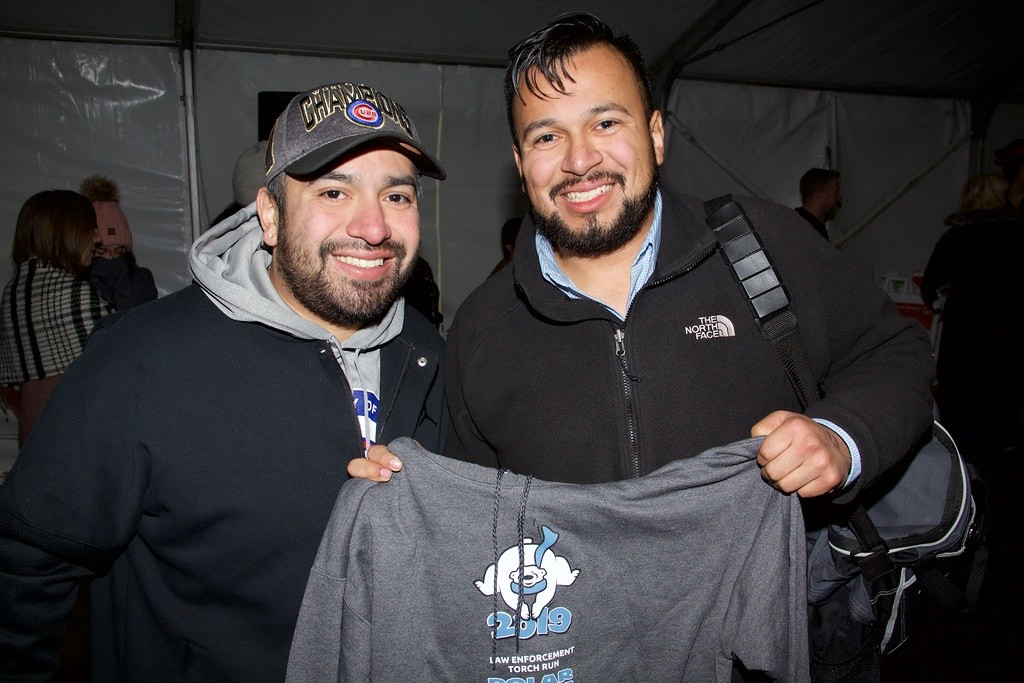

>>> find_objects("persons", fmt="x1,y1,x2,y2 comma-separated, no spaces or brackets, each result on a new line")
207,141,270,226
794,166,844,242
0,79,448,683
994,137,1024,208
920,173,1024,494
405,253,444,332
0,191,119,458
345,10,939,683
82,175,159,315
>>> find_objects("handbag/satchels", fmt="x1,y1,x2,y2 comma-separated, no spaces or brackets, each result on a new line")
704,193,986,659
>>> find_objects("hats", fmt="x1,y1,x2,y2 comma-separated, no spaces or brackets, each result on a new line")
80,176,134,249
263,80,447,189
231,140,269,206
